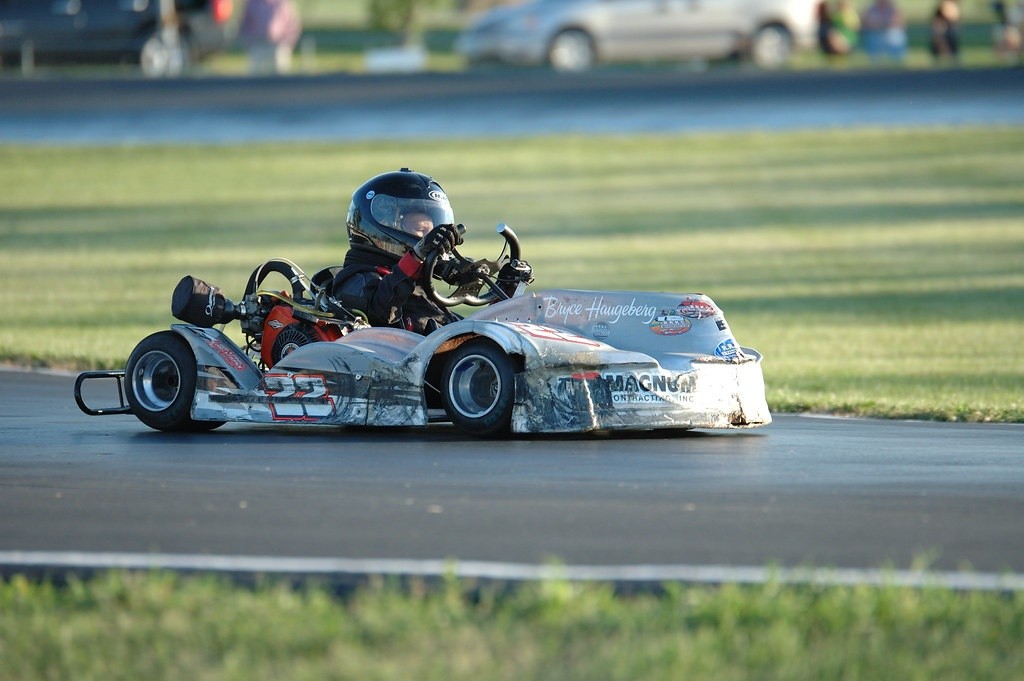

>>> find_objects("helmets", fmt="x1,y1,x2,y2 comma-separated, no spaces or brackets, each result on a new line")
345,168,455,259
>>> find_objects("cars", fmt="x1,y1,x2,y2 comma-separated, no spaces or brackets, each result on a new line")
0,0,233,81
454,1,818,74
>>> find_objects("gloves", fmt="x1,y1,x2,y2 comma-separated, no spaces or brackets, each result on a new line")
412,224,465,262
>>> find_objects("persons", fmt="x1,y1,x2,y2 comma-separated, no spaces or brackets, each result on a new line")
231,0,306,77
327,165,466,335
814,0,1018,65
